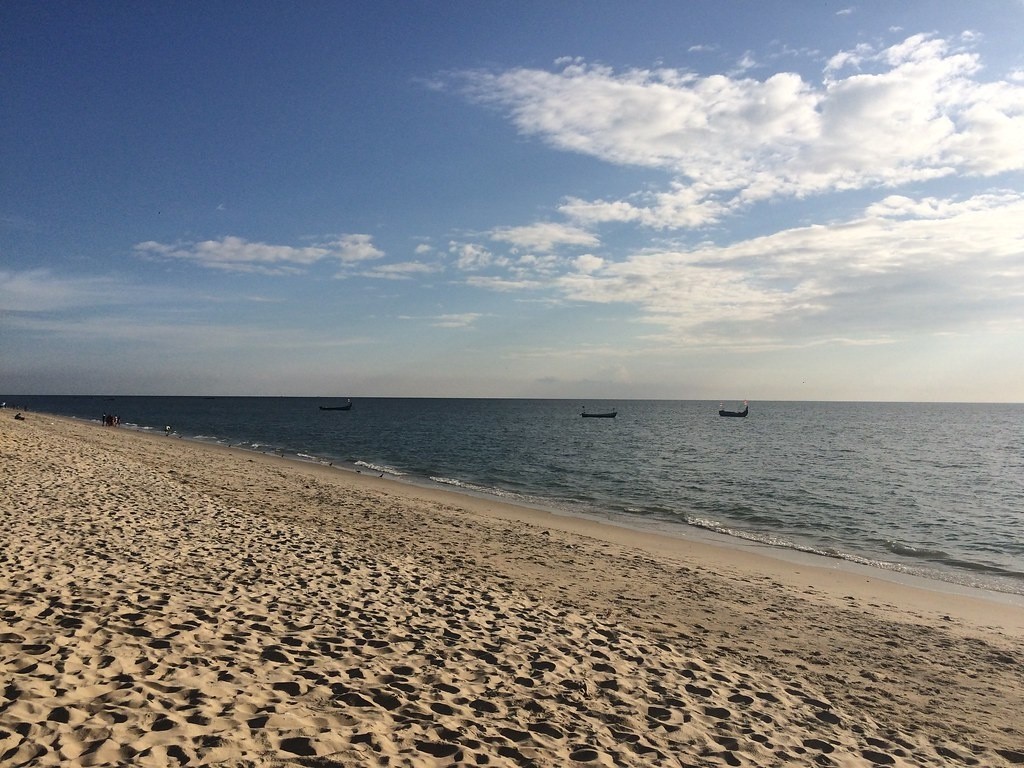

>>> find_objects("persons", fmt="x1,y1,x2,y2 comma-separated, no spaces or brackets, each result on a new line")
4,403,27,411
14,412,21,419
164,425,171,436
102,413,120,427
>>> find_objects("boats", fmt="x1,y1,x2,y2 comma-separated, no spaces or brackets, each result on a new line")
582,412,617,418
318,402,352,410
719,406,748,417
